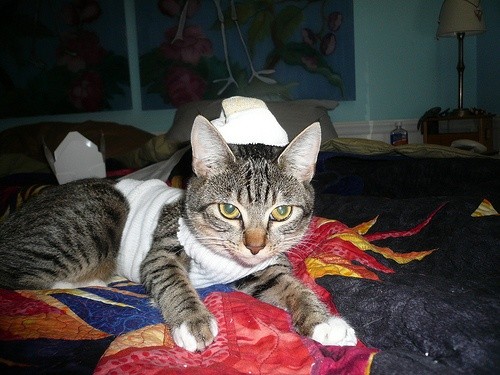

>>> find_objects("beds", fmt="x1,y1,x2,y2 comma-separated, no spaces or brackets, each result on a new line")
0,119,500,375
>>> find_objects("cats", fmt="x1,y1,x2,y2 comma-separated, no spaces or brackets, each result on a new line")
0,114,356,353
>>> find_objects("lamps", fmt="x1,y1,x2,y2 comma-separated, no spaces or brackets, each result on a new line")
435,0,487,118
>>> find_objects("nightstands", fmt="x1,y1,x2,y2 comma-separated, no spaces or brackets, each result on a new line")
418,114,494,155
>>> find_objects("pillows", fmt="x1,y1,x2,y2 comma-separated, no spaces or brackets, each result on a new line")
171,99,341,151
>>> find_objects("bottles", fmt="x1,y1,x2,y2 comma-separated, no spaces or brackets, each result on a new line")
391,121,408,145
